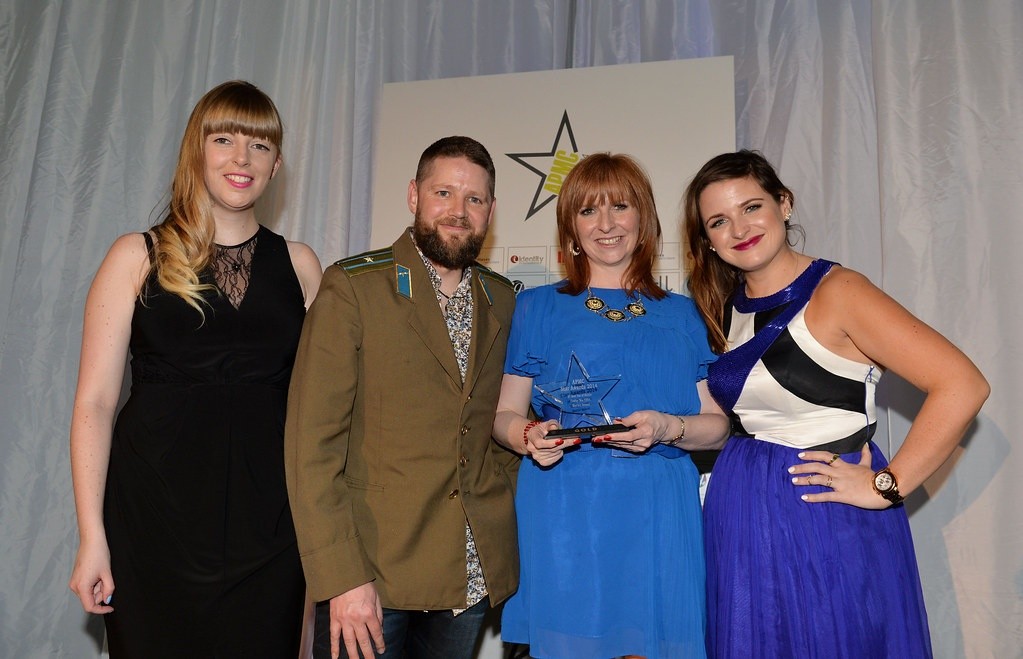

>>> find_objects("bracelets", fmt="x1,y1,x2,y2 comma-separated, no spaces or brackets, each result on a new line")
524,421,543,445
663,417,684,446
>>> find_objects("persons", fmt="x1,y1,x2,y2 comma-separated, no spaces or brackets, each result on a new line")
69,80,322,658
285,136,520,658
687,149,990,658
492,153,733,659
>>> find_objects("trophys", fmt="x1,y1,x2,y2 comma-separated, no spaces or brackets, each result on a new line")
533,350,636,440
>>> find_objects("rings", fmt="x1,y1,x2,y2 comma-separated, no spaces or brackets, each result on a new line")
828,454,840,464
632,441,634,445
806,475,813,486
826,476,832,487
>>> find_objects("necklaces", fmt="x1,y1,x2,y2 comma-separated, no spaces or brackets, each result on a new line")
438,270,463,311
585,286,646,321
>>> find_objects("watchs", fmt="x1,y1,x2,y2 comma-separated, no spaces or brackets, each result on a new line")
871,467,903,504
